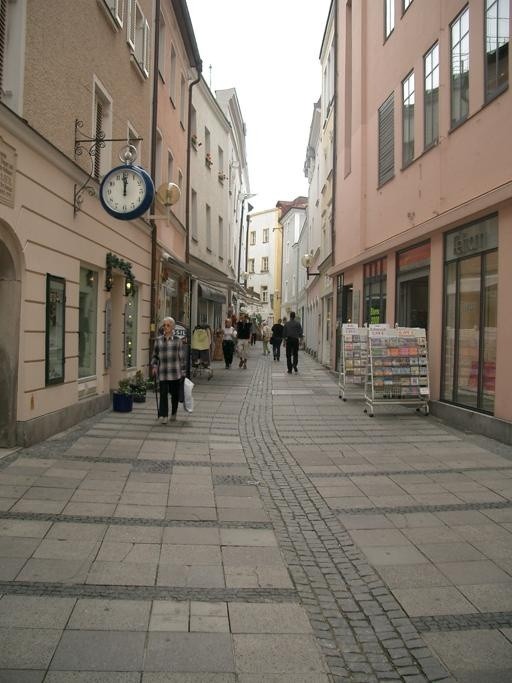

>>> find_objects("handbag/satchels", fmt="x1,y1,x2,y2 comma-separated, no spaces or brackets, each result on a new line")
179,378,184,402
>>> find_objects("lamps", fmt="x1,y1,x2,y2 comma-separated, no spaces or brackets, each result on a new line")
144,182,182,227
241,272,249,280
301,253,320,280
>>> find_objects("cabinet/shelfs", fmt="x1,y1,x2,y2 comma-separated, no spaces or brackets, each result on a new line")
338,323,399,402
363,322,431,417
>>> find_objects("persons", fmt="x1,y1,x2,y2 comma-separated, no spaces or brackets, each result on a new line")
250,318,287,361
149,316,187,424
234,315,253,369
281,312,303,373
221,318,237,369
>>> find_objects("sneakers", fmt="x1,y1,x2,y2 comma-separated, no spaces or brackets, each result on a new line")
287,365,298,373
239,359,247,369
159,413,176,424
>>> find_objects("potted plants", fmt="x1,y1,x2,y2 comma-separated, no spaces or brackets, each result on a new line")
128,369,147,403
113,377,132,411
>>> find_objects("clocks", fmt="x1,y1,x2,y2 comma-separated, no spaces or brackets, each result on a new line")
100,138,154,220
